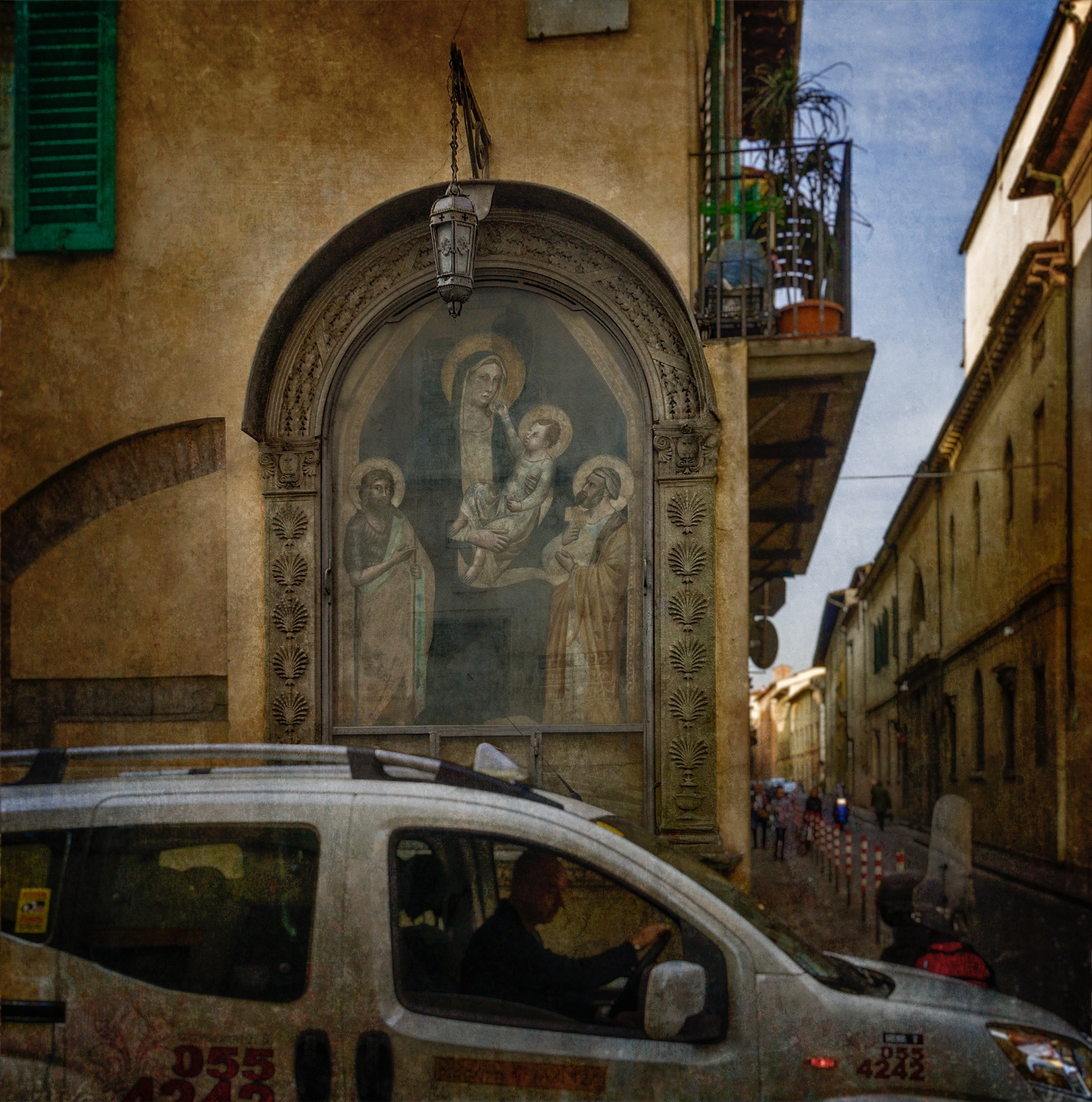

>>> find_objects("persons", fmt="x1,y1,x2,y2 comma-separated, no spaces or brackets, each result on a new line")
460,849,673,1028
751,777,996,993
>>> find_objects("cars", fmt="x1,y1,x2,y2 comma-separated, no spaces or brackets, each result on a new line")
770,777,797,792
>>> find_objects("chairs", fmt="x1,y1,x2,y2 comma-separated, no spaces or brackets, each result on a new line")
397,855,462,993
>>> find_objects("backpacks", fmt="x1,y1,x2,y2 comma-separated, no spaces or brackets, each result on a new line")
916,943,987,988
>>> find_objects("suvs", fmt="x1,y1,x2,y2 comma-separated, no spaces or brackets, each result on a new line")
0,743,1092,1102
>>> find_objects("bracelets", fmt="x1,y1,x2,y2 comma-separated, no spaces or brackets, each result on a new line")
631,941,641,951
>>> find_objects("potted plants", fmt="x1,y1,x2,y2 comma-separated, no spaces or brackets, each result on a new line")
745,59,854,337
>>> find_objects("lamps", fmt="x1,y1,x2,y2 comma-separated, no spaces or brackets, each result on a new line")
429,39,480,319
